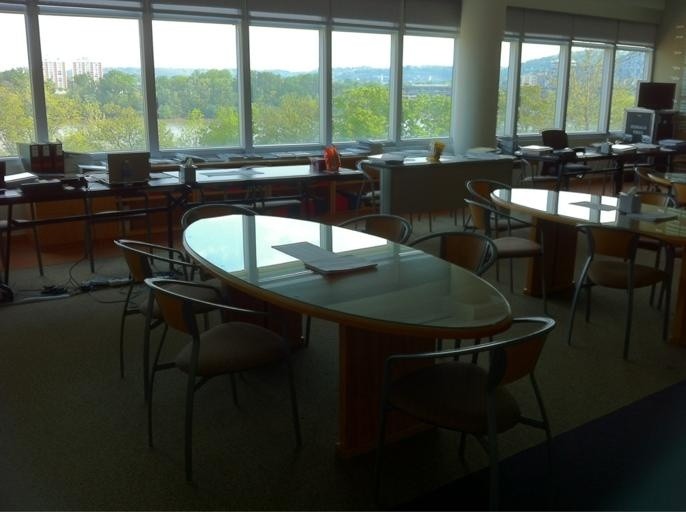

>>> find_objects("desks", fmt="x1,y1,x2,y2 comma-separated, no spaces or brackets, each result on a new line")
1,162,365,296
182,213,513,461
513,129,684,197
355,152,514,230
489,187,684,347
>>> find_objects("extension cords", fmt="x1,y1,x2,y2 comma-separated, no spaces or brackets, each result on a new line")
108,277,133,285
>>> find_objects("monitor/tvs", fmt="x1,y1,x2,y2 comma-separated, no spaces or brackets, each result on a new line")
635,80,681,111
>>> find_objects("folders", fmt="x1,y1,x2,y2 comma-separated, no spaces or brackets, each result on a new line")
30,144,64,173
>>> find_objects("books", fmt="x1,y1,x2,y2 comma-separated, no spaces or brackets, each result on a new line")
149,138,384,165
626,212,678,223
271,241,377,276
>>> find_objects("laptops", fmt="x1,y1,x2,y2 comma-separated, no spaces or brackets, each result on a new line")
100,152,150,185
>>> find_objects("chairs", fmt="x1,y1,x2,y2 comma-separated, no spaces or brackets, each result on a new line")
673,183,684,208
375,315,557,512
181,204,258,229
633,191,678,211
143,276,302,485
463,197,548,315
466,178,531,233
405,230,498,361
336,213,412,245
567,222,676,361
114,239,203,381
